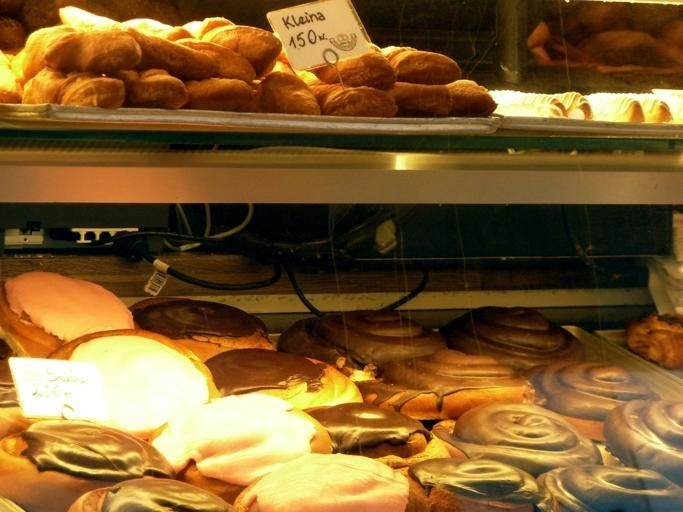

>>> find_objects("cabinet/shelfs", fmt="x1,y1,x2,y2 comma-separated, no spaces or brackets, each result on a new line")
0,0,683,512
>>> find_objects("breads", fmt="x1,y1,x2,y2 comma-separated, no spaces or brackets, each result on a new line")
301,403,434,457
58,5,125,29
625,313,683,371
393,81,451,117
277,309,448,378
531,355,662,423
449,78,498,118
129,33,218,81
582,27,660,67
21,67,64,105
175,39,255,82
0,430,180,512
200,16,235,37
204,26,285,73
533,465,683,512
607,397,683,486
127,72,191,109
8,27,141,76
259,74,322,115
357,347,535,419
407,457,542,512
189,78,260,111
414,304,587,374
380,46,465,85
310,82,398,117
65,474,233,512
273,31,399,88
129,295,277,365
204,348,364,408
231,453,409,512
60,76,129,110
21,421,177,481
42,329,220,435
0,269,140,359
0,495,26,512
0,51,25,104
187,396,334,486
168,26,192,38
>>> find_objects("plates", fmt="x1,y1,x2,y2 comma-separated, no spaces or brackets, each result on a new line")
561,326,683,415
1,104,502,138
501,116,682,140
593,328,683,387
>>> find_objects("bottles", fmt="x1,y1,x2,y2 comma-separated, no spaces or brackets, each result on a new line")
433,402,605,475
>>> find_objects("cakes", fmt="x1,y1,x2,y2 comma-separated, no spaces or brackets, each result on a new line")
586,92,642,121
489,90,593,119
639,94,673,121
